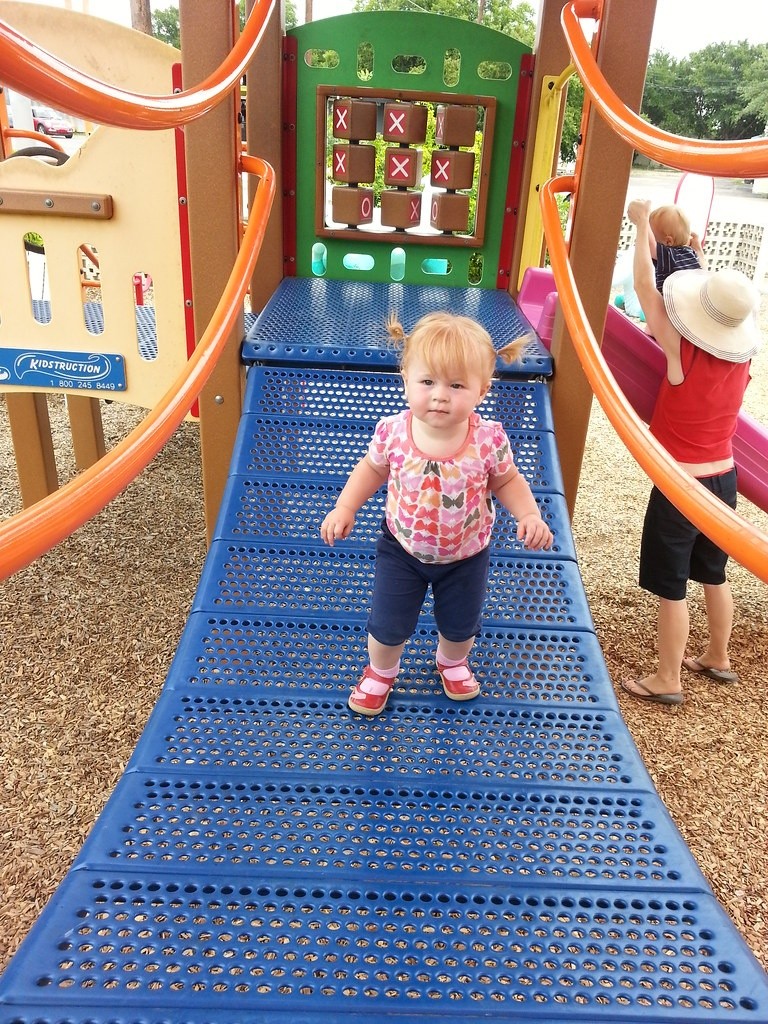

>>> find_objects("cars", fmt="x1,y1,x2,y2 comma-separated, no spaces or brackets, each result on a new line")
31,106,74,139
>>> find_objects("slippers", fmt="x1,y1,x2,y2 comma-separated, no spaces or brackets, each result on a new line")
682,659,738,682
621,676,684,704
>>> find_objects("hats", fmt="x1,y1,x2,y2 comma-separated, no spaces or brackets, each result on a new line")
662,268,761,363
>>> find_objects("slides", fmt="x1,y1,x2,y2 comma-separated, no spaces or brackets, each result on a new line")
516,265,768,513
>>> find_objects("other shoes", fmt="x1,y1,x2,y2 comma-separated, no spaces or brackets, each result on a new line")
348,665,396,716
435,658,480,701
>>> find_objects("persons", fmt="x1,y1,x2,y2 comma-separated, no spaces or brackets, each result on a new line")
319,307,555,716
645,204,705,347
626,199,757,704
